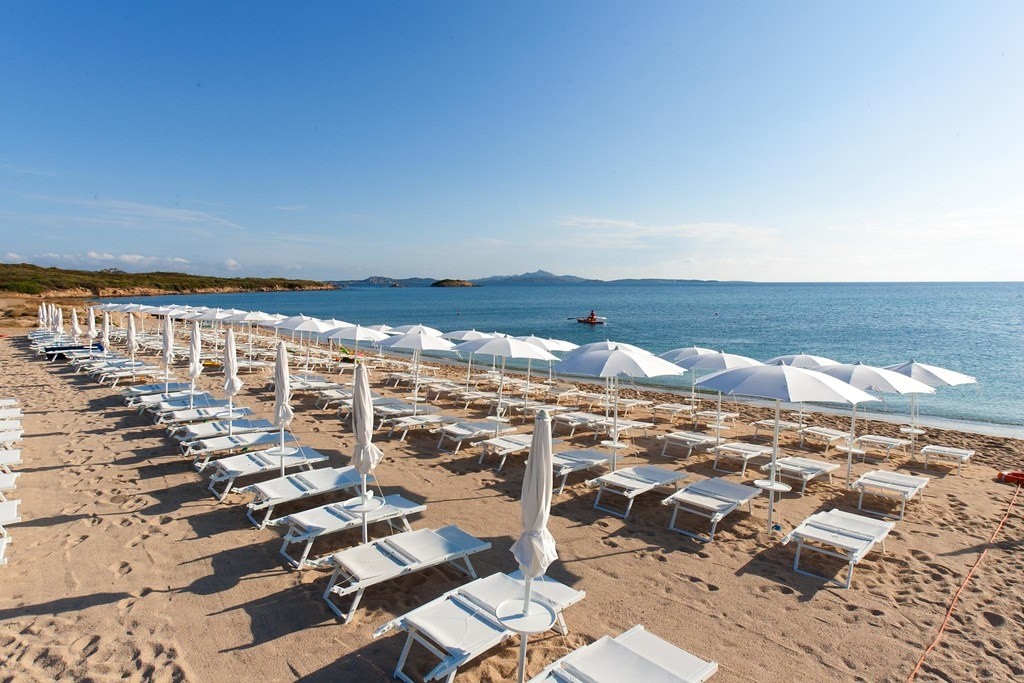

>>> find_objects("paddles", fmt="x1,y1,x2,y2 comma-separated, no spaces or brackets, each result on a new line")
567,316,607,320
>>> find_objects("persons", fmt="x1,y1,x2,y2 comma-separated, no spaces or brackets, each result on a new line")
587,310,597,322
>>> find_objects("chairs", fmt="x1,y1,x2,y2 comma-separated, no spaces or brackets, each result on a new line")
0,420,23,432
0,470,21,501
0,398,18,409
920,445,975,476
760,457,840,497
0,429,24,450
0,408,24,421
750,420,807,442
855,435,912,462
28,325,781,683
782,508,897,590
0,531,12,567
796,426,855,453
0,498,22,535
851,469,930,520
0,448,23,473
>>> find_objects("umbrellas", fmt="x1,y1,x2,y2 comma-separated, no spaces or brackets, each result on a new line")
274,342,294,477
71,308,82,346
223,328,242,435
88,302,977,534
350,362,384,543
37,302,63,347
509,410,559,683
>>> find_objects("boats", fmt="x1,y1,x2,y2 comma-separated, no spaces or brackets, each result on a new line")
577,317,605,325
